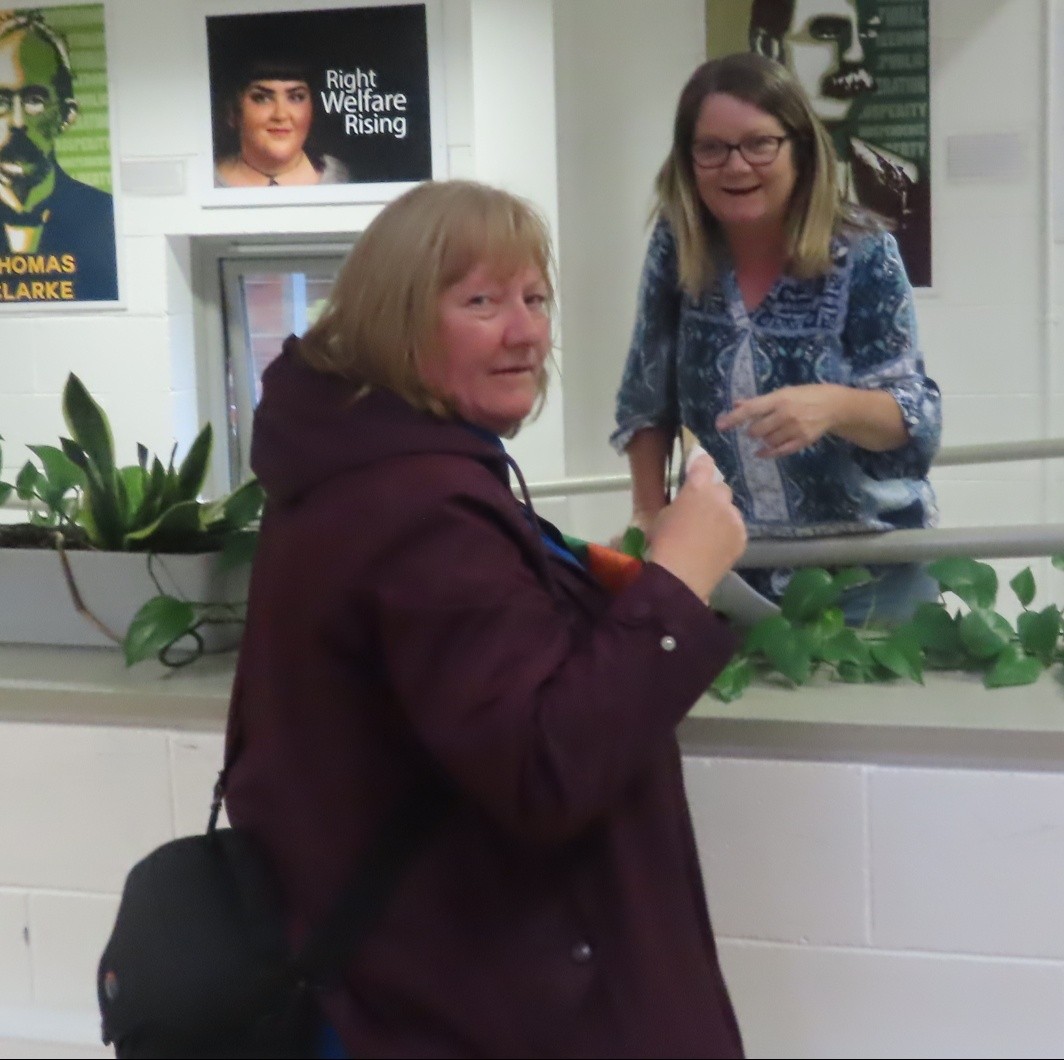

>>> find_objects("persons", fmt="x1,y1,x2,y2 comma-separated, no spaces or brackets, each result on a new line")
213,38,355,187
608,54,946,600
224,187,748,1060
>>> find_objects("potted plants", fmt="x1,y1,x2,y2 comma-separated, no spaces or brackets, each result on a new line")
0,372,1064,702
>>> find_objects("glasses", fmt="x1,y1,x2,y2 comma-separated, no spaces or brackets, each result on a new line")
693,133,791,168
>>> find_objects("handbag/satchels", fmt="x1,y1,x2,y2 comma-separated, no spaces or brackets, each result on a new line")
97,830,337,1060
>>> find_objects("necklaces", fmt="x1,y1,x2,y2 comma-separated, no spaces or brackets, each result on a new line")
239,150,304,186
